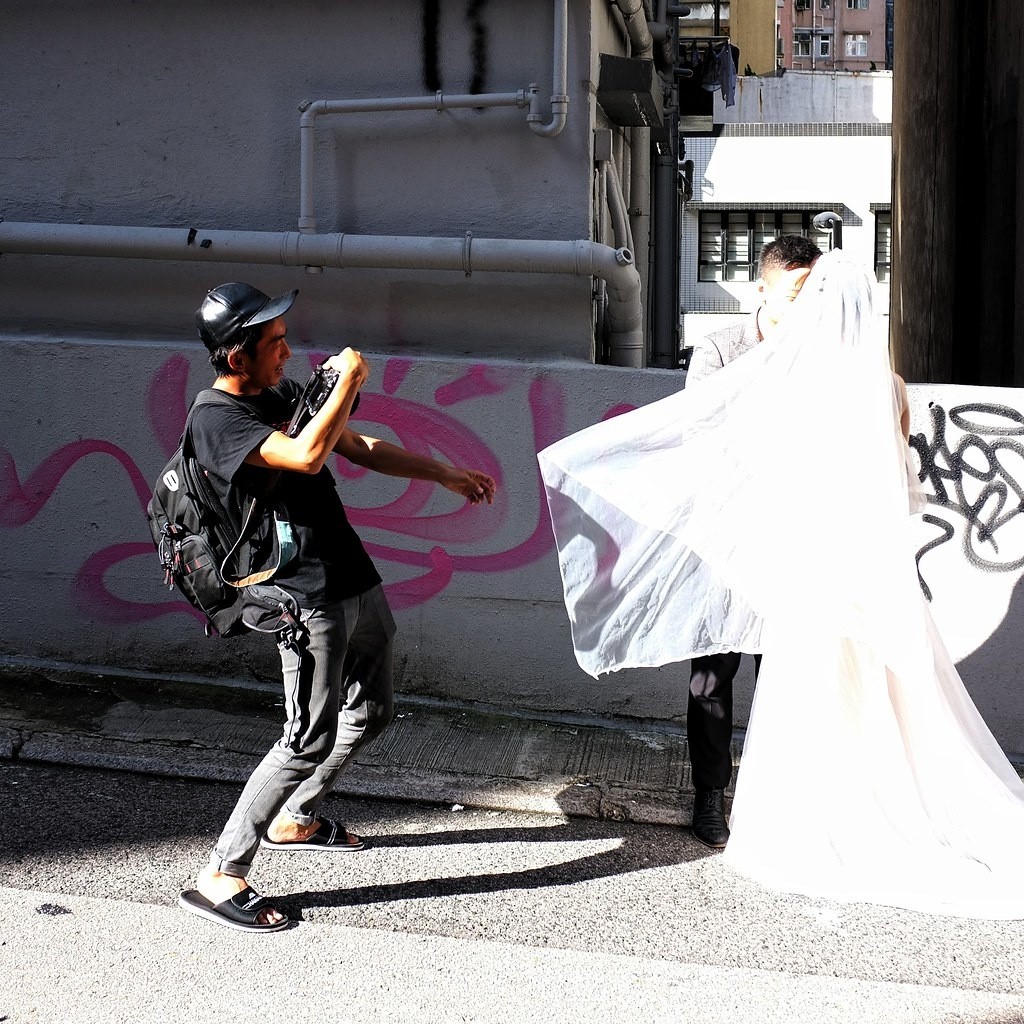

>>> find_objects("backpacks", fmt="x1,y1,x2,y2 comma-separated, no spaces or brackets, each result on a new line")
145,387,299,640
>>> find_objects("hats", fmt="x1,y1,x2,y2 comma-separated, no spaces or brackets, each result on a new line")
194,282,299,352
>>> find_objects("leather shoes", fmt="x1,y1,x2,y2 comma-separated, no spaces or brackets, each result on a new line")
692,787,731,848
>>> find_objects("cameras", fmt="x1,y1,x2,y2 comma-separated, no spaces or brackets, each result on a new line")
304,361,361,417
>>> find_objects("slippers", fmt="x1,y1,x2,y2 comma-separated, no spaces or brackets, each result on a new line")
260,815,365,852
179,886,289,932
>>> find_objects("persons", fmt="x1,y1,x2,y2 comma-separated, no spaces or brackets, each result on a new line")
177,282,497,935
684,234,909,848
